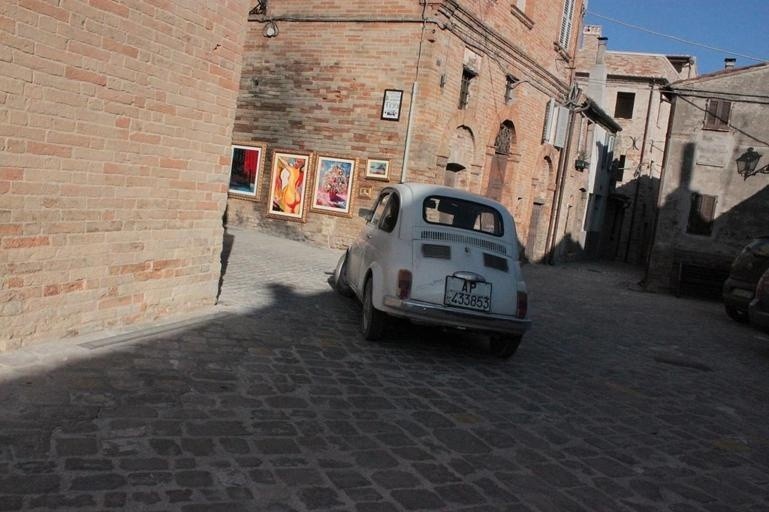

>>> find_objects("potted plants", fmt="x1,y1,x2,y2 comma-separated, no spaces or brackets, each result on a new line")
576,148,592,163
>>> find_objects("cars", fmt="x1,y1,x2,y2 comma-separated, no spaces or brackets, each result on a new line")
718,235,769,337
326,182,535,359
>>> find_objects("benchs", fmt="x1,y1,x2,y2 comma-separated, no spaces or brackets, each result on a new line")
662,242,735,299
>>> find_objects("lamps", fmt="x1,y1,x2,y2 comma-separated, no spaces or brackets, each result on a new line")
735,145,769,182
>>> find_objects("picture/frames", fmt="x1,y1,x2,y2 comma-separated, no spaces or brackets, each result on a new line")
226,138,268,204
262,146,313,224
309,149,360,219
363,155,392,183
379,88,405,122
